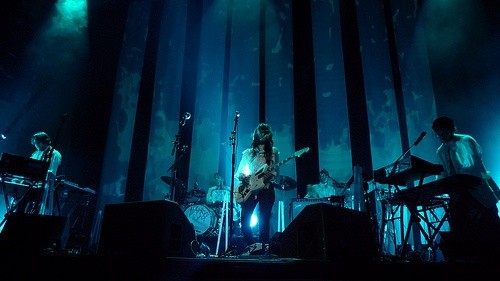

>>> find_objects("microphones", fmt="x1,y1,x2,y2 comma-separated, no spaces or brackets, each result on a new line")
180,112,191,127
415,132,426,146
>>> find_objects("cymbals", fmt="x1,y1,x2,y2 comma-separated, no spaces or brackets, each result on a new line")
272,175,297,191
161,175,171,186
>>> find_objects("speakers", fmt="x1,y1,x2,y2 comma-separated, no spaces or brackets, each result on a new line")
18,183,96,256
98,200,196,257
0,213,67,253
438,219,500,262
269,203,372,263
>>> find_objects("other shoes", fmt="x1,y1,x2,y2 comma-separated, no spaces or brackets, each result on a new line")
242,251,250,256
265,244,269,251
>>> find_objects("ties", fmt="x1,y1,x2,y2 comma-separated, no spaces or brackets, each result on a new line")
446,146,456,176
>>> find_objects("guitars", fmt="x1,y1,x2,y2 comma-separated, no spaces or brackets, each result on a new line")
235,148,309,204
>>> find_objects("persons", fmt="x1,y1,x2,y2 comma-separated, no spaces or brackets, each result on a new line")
431,117,500,199
235,123,280,255
303,170,335,198
12,133,61,215
205,173,236,235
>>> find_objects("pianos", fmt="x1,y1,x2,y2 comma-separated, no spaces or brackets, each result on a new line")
373,155,486,269
0,152,95,233
289,196,345,222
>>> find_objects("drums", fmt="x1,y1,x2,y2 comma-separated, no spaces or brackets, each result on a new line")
209,188,235,208
183,204,217,234
233,205,240,221
